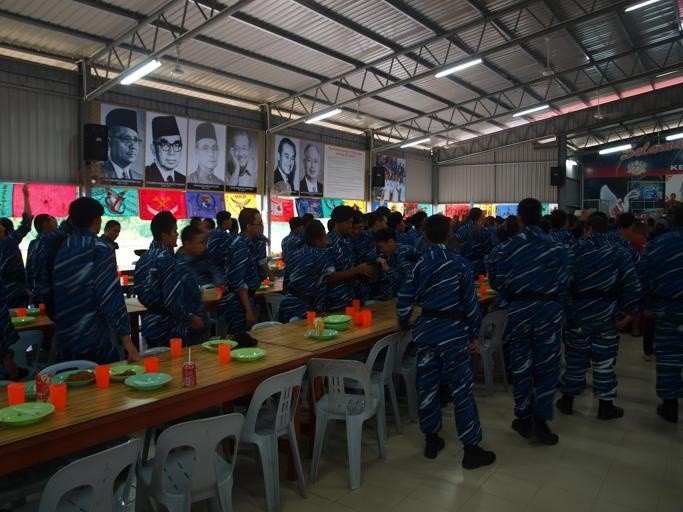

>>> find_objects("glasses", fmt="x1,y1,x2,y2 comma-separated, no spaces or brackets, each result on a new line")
157,140,183,152
114,135,141,145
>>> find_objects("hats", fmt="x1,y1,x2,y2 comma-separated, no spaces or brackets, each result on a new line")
104,108,139,136
194,122,217,147
151,115,180,138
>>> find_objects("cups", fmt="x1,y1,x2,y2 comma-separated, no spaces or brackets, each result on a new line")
263,278,269,287
169,337,181,356
306,310,315,327
49,383,66,411
344,299,371,328
143,356,158,372
95,365,110,388
277,252,283,268
17,307,26,317
117,271,128,284
7,383,25,405
38,303,45,316
217,343,230,363
314,318,324,335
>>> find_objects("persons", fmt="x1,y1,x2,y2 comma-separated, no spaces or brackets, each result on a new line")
185,121,224,186
371,153,407,202
98,108,144,181
145,114,185,183
225,129,258,187
0,196,427,385
299,143,323,193
271,136,298,191
395,197,682,471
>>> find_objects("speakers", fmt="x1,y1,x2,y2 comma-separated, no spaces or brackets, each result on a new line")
551,167,564,185
372,167,385,186
83,124,108,161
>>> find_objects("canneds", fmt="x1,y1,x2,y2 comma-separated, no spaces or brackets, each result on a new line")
183,362,196,387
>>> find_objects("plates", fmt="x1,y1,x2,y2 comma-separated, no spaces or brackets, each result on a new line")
24,378,59,401
26,308,38,316
10,316,35,324
307,328,337,340
202,339,238,351
256,282,273,291
324,315,351,333
473,276,496,298
0,402,54,427
229,346,266,362
123,373,171,392
53,369,96,386
109,365,145,381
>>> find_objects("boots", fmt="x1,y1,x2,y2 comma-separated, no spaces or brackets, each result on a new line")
598,398,624,419
657,398,678,423
512,415,532,439
424,432,444,458
556,395,574,414
462,445,496,469
533,416,559,444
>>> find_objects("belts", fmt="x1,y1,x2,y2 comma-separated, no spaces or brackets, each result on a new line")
575,291,615,299
228,286,257,297
509,293,557,302
422,308,466,320
288,287,313,299
146,303,167,315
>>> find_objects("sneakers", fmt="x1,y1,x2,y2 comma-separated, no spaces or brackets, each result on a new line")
643,351,655,362
